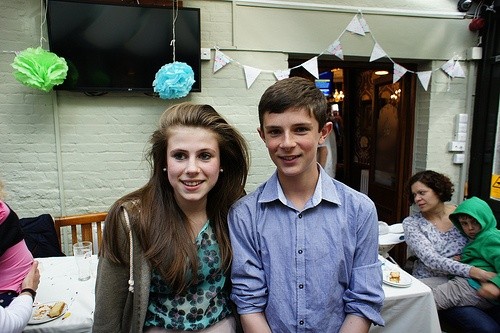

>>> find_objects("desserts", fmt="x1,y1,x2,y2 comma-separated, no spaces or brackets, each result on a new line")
48,301,65,317
389,271,401,282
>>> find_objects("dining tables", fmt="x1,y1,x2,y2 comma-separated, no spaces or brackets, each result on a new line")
29,255,442,333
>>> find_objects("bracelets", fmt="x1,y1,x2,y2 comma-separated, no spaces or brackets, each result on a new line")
21,288,36,303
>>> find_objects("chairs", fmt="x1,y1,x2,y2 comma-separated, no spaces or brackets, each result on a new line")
55,212,107,255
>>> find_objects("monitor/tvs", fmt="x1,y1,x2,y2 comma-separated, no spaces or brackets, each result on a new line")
44,0,201,93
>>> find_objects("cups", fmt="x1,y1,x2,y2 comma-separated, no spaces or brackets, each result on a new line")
73,241,92,281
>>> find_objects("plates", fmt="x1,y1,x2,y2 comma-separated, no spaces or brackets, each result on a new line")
27,300,68,325
383,268,412,287
388,224,404,233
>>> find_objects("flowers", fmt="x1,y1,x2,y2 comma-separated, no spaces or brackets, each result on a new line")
11,48,69,93
152,61,196,99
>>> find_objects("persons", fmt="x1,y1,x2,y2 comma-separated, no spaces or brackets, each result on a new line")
227,76,384,333
0,195,37,308
0,259,40,333
91,102,251,333
416,196,500,311
316,128,337,179
402,170,500,333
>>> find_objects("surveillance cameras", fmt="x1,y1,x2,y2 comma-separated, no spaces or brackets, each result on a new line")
458,0,473,12
